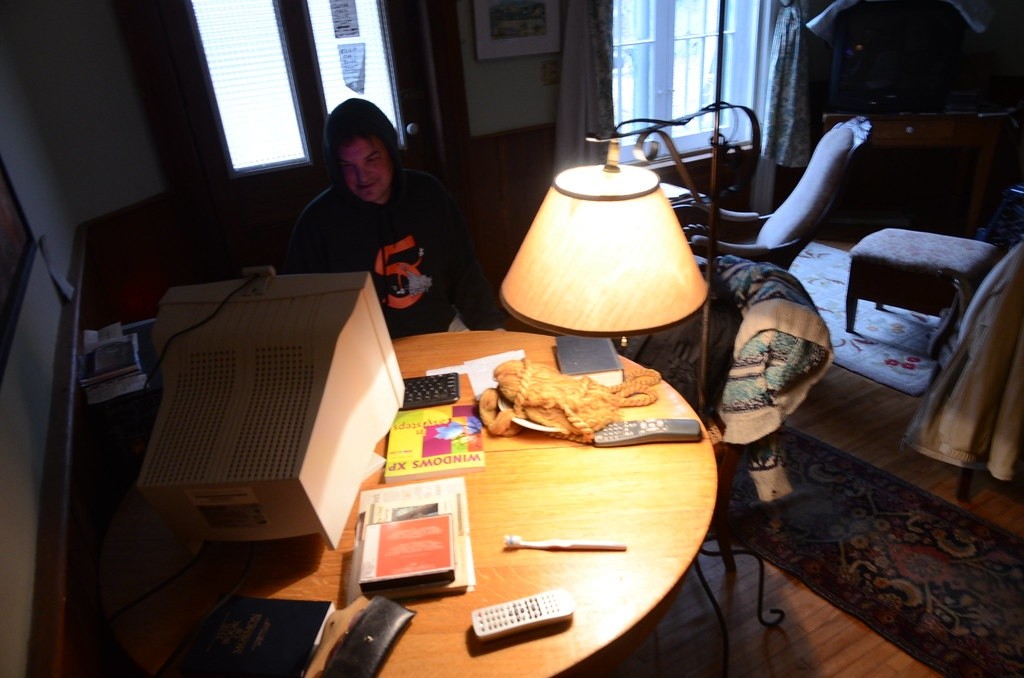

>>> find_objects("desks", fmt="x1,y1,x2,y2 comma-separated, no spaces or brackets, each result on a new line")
821,109,1009,238
93,326,719,678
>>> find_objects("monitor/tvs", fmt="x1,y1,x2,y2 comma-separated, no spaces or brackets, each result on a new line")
135,271,408,557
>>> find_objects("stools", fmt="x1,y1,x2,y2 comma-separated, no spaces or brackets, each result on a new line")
846,229,996,357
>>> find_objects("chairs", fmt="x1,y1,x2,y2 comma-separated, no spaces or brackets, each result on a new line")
623,253,831,570
676,113,873,271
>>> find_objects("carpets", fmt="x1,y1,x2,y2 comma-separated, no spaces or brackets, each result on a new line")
789,243,965,395
716,422,1024,678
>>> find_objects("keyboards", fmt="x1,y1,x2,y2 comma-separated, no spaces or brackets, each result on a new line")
400,372,460,412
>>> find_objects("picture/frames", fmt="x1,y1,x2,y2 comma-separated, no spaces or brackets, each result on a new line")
0,149,37,387
473,0,562,62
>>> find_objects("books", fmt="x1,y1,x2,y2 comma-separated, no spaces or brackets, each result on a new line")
78,333,141,387
346,476,477,605
155,590,335,678
555,335,625,387
385,373,485,483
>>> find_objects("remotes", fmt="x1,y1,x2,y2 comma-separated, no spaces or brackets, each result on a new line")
470,589,576,641
593,418,703,448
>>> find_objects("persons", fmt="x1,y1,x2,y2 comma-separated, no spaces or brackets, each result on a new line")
285,98,506,341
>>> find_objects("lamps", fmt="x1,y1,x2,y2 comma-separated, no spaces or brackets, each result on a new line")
498,101,785,678
